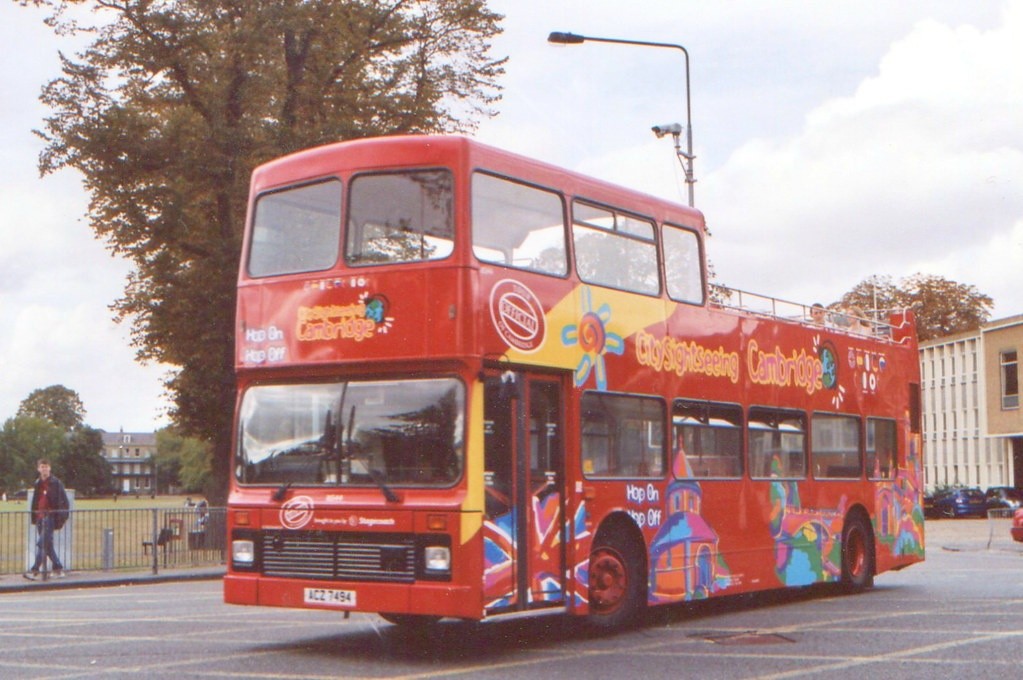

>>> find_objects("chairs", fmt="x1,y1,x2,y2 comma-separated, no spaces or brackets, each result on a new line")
142,526,173,555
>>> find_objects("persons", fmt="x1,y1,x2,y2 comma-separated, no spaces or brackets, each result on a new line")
323,406,390,484
22,458,70,580
806,304,876,341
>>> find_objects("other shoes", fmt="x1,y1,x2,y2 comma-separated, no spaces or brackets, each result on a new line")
24,571,39,580
48,570,66,578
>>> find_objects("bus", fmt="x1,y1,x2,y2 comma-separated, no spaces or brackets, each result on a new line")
220,134,930,629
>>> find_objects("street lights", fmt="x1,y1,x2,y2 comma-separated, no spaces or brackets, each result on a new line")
548,32,719,206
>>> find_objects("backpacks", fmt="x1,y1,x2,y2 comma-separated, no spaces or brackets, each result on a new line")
54,479,69,529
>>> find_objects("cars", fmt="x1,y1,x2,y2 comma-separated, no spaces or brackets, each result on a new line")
987,486,1023,517
934,485,991,519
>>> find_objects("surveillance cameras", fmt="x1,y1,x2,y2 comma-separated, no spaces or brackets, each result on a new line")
651,124,667,137
